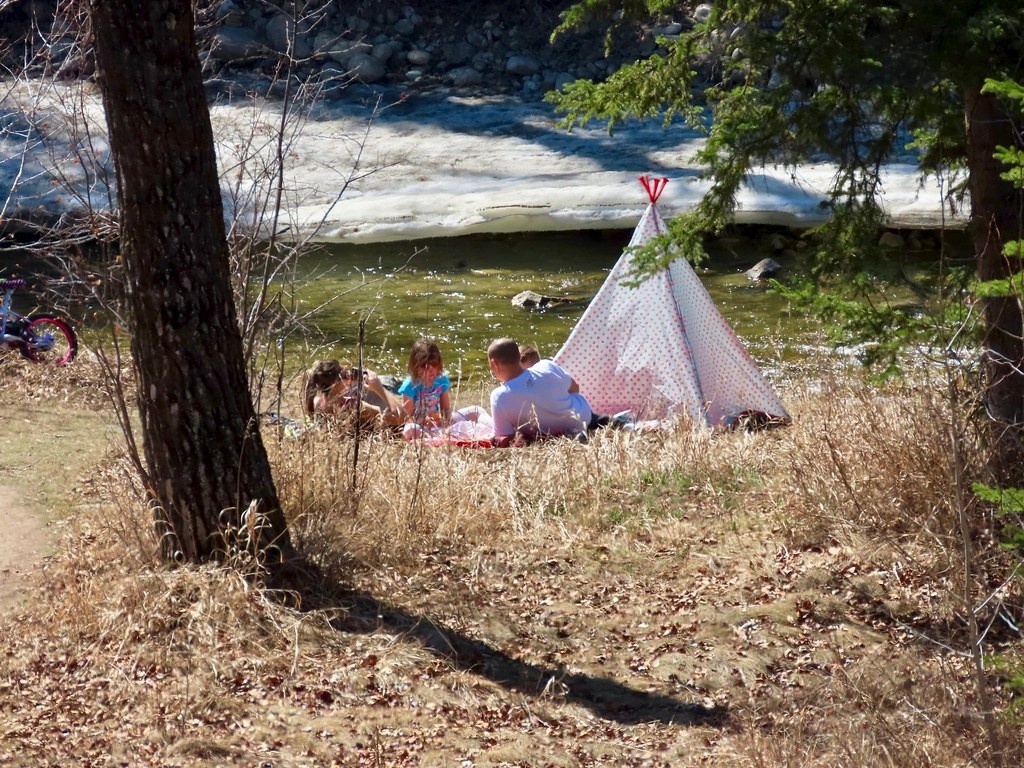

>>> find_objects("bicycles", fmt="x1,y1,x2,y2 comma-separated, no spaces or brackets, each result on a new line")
0,278,79,370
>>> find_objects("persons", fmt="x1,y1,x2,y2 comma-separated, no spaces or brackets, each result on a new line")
488,339,640,447
302,359,404,431
398,340,492,445
519,345,540,369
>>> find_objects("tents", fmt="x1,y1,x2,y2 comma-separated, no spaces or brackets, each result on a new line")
554,176,792,442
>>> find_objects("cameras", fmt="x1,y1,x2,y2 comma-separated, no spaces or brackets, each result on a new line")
352,367,368,382
